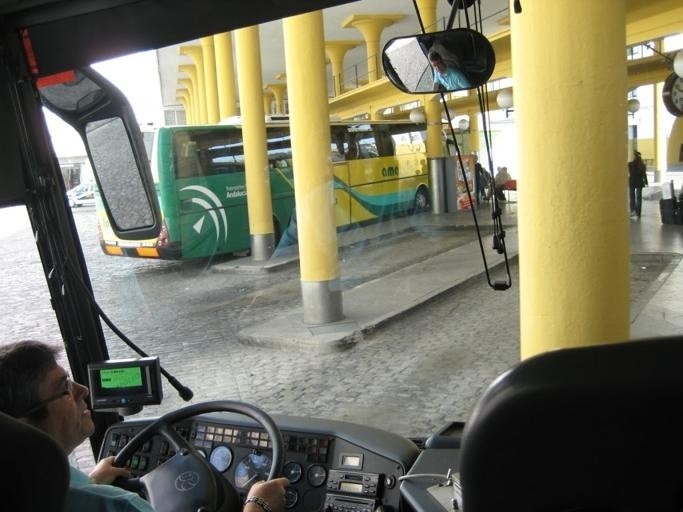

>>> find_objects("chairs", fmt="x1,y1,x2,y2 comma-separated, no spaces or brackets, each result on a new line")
0,413,72,512
457,334,682,512
500,180,516,203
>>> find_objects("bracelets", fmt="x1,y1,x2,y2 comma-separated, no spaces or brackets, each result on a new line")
245,497,273,512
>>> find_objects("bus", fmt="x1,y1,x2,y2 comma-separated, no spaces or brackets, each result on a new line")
93,114,458,260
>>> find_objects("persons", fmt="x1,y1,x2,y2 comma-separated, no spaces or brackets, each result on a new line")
629,152,648,220
427,51,471,91
476,155,511,205
0,341,290,512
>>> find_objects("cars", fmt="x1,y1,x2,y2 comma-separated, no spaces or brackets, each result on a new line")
64,178,95,207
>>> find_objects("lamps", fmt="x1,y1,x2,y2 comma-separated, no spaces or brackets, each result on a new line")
672,49,682,78
458,119,469,130
409,109,426,125
627,99,640,119
440,109,455,121
496,86,513,118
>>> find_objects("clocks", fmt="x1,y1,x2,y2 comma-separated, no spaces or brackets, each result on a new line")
662,71,682,117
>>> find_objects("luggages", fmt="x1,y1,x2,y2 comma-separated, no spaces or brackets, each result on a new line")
659,182,682,225
